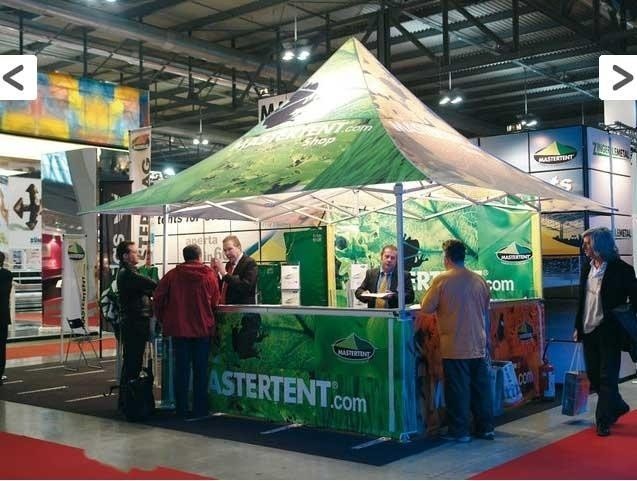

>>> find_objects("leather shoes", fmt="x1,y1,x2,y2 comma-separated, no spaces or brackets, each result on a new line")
594,400,633,438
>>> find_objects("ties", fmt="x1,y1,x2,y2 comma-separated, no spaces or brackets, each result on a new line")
221,262,233,305
374,272,389,309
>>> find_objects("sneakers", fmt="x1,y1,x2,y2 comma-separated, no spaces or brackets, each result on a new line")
473,428,496,441
168,410,211,421
439,432,472,445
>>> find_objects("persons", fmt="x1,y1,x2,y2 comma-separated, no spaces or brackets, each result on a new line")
573,225,637,435
422,238,497,442
210,235,258,304
0,251,13,380
136,249,159,282
356,244,415,308
115,241,157,421
151,244,221,419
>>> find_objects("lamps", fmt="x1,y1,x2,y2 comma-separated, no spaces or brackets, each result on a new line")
507,67,540,133
192,85,209,145
439,35,465,105
280,1,313,60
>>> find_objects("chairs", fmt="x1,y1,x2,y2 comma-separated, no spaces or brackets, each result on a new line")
63,316,104,372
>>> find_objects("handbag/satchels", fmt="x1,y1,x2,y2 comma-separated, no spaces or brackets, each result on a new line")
560,368,592,419
480,356,527,420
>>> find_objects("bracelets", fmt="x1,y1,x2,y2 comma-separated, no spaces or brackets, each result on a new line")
223,271,228,276
